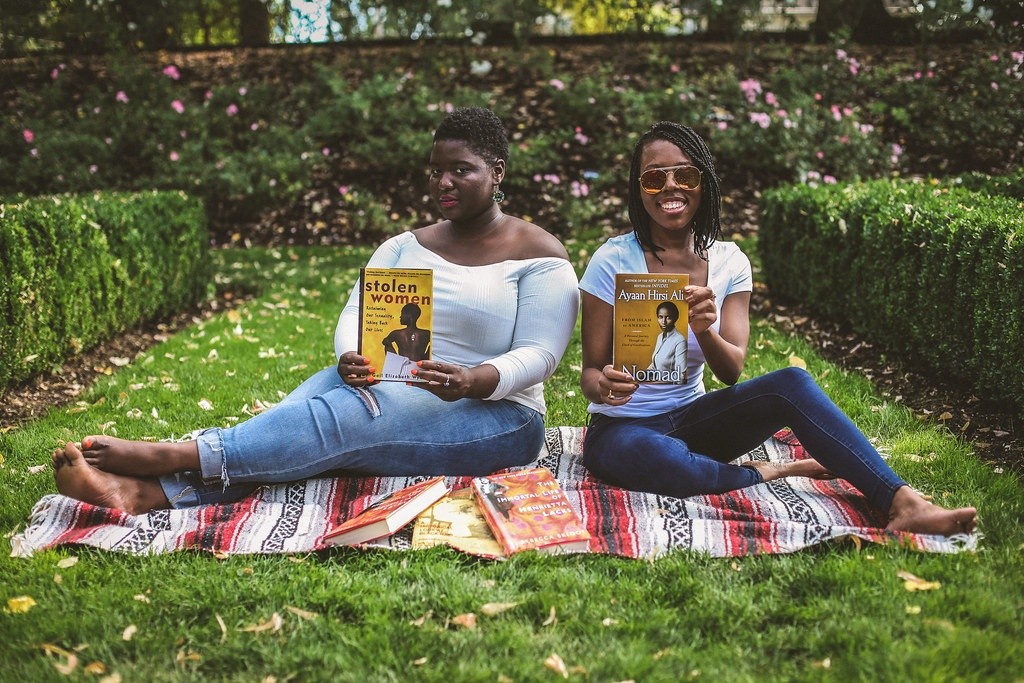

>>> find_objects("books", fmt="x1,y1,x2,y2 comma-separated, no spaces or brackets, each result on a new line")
325,468,589,559
358,267,433,383
613,273,689,385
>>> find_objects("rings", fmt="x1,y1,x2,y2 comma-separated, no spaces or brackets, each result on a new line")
711,293,716,300
444,375,449,386
608,390,615,399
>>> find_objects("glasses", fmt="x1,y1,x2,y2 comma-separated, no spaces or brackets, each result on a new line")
638,167,704,195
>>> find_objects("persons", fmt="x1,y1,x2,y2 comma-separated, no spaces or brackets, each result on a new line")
53,106,578,516
578,122,978,537
638,302,687,385
382,303,430,362
